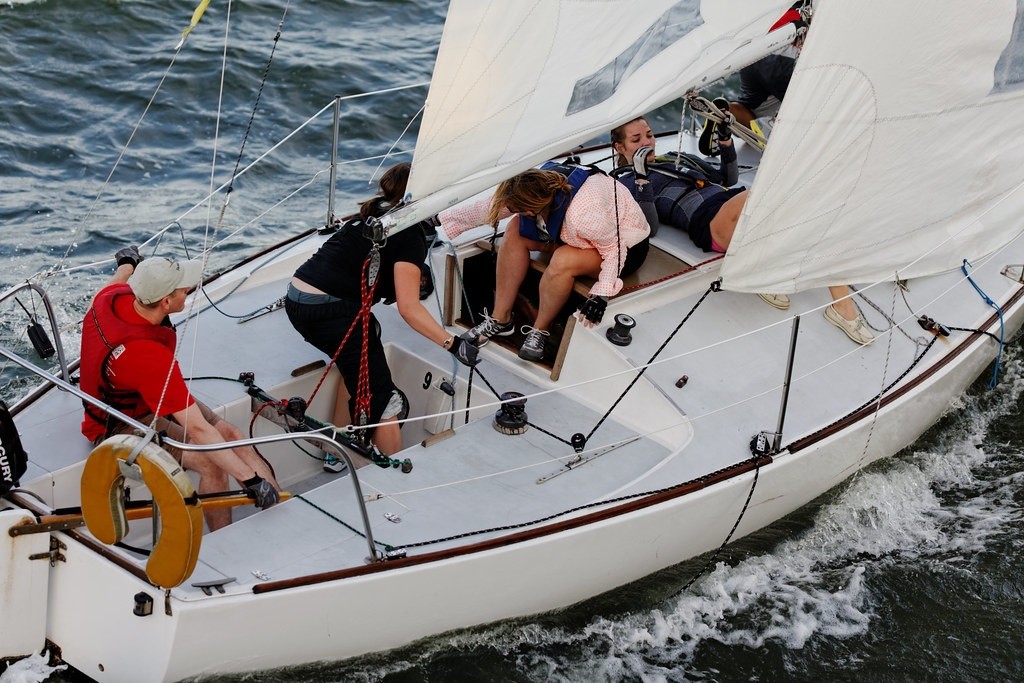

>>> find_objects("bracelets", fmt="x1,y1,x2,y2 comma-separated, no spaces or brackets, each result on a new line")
443,337,453,349
635,180,650,191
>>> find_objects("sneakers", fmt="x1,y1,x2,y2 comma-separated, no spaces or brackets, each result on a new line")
749,115,775,149
698,97,733,157
757,292,790,309
323,453,345,473
460,307,514,348
521,325,551,360
824,305,874,346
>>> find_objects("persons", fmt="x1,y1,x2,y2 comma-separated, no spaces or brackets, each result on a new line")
698,0,809,157
285,164,481,474
459,160,652,361
80,246,282,533
609,110,876,346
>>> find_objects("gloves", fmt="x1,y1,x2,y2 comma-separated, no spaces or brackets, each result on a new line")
114,245,145,274
715,110,738,141
242,472,280,511
447,336,481,367
631,146,654,181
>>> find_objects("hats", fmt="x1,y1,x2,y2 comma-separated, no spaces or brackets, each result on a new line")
127,257,202,305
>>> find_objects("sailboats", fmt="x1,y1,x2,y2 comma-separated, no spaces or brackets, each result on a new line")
0,0,1024,683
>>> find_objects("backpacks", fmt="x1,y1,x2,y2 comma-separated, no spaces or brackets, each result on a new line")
0,399,28,496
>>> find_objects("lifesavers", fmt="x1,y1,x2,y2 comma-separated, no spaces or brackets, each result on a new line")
77,432,204,590
